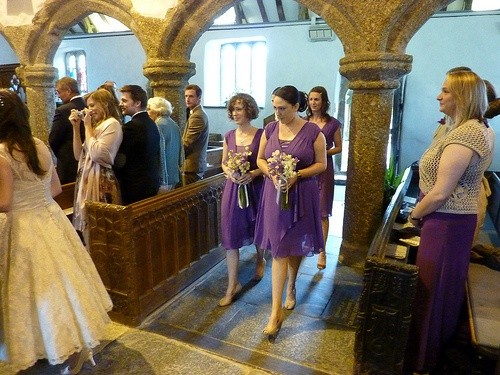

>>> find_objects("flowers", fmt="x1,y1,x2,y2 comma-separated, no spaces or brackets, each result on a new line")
267,149,301,212
223,145,253,210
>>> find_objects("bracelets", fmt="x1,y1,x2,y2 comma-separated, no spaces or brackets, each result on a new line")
296,170,302,180
410,211,423,221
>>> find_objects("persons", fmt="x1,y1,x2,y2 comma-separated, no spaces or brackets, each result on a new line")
68,88,124,250
302,86,342,270
182,84,210,187
263,87,281,129
471,80,500,249
112,85,162,206
0,88,114,375
49,76,88,184
255,85,327,336
218,93,264,306
97,85,123,125
146,97,185,194
406,66,492,375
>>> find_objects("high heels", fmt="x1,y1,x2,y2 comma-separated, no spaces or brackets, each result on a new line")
317,254,326,270
285,288,296,310
219,282,242,306
254,259,266,281
263,313,282,335
61,347,95,375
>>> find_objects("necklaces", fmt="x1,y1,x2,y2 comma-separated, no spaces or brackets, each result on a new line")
237,126,254,143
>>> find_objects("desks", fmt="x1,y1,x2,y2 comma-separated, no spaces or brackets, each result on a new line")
352,163,420,375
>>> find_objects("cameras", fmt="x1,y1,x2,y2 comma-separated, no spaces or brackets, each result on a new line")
76,110,83,120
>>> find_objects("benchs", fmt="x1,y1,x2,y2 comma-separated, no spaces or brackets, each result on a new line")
52,182,75,216
465,172,500,357
208,132,223,147
86,172,227,329
207,145,223,169
